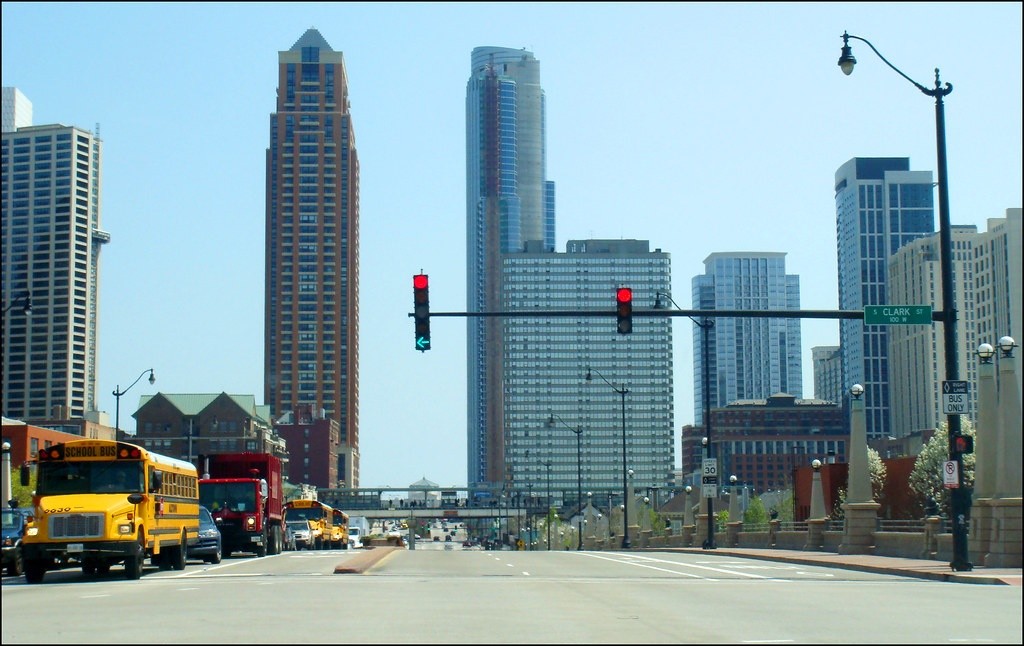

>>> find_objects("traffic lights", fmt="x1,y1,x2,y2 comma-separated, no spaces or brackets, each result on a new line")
617,288,633,334
413,274,431,350
955,434,973,454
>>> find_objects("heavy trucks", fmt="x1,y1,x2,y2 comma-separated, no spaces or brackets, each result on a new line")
196,451,285,558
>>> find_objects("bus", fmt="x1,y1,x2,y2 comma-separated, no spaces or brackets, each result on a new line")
21,439,199,583
286,519,315,550
282,500,350,550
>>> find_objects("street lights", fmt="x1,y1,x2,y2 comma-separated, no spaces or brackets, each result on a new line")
549,412,584,552
112,367,156,441
585,367,632,548
836,29,973,571
652,291,718,549
537,458,552,552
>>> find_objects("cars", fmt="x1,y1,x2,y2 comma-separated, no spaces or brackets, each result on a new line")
187,503,224,565
388,520,421,546
286,525,297,551
432,519,503,551
1,508,34,577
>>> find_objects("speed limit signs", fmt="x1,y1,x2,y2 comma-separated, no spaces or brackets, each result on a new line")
703,459,717,477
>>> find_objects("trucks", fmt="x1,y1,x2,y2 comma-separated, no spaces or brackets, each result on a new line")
349,516,369,547
